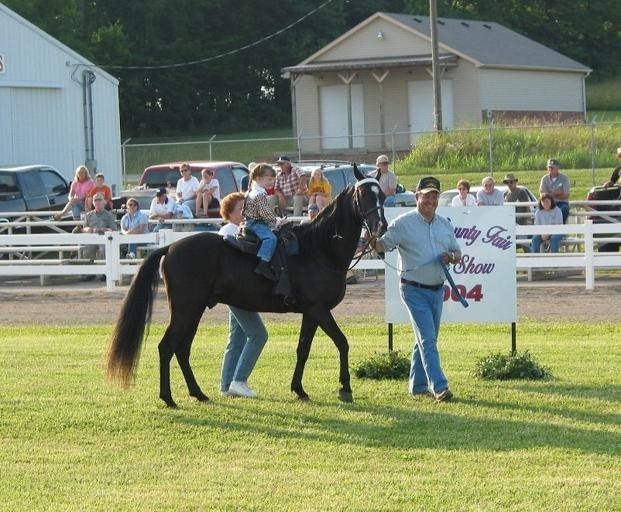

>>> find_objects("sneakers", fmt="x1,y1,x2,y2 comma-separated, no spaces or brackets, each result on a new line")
254,260,276,280
411,390,434,398
222,381,258,398
435,389,453,402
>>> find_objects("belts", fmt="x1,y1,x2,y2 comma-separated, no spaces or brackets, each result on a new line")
400,277,445,290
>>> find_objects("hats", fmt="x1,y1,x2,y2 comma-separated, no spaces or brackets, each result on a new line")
93,192,103,202
420,176,440,196
277,156,291,164
546,159,558,168
376,155,391,165
503,174,518,183
155,187,167,197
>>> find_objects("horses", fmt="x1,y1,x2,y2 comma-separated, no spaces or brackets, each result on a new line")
108,163,386,409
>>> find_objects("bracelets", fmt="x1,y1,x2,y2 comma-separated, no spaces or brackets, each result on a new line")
451,251,455,263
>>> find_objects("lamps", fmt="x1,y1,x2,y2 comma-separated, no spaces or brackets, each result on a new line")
377,31,383,40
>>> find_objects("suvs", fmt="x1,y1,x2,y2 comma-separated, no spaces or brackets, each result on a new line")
270,160,420,211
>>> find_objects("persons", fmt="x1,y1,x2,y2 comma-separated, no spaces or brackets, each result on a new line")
363,176,462,403
604,149,621,187
50,163,221,282
240,154,332,279
218,191,268,400
370,153,397,206
452,157,570,255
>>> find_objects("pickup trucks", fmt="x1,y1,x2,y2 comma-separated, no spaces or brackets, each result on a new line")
112,160,274,238
0,163,72,259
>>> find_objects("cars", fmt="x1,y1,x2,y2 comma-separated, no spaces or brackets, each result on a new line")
438,184,551,252
585,184,621,253
